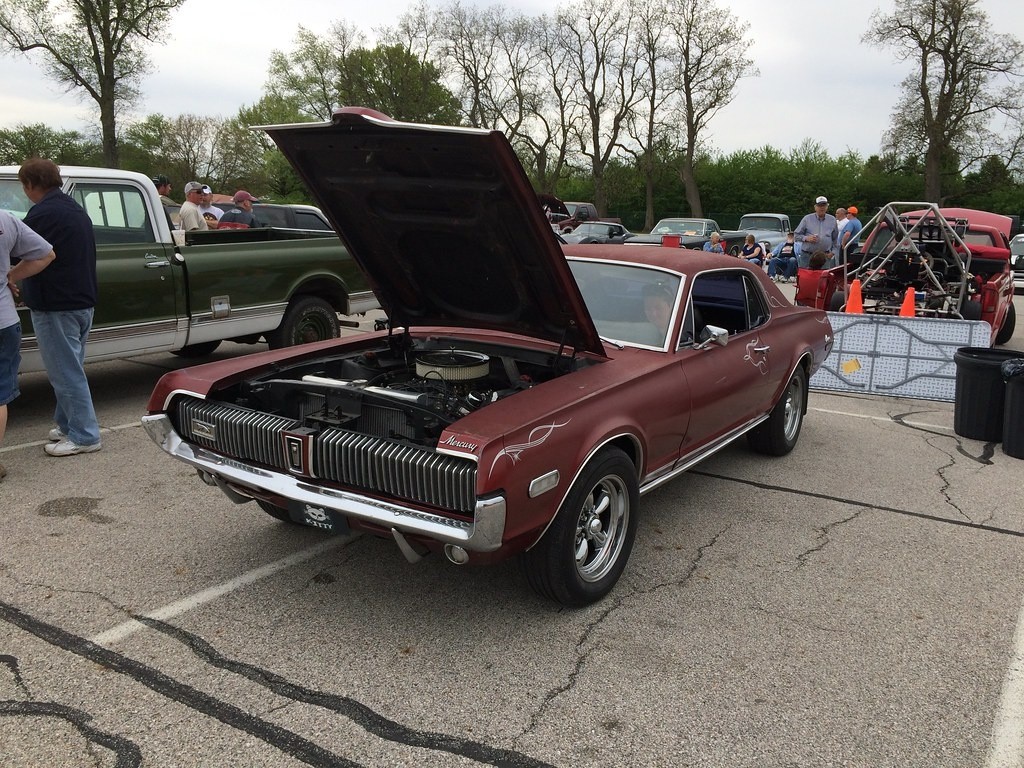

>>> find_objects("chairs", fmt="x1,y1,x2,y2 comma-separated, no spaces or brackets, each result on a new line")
660,236,798,283
793,267,837,310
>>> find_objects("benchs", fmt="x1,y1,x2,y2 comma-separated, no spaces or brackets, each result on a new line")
608,294,747,334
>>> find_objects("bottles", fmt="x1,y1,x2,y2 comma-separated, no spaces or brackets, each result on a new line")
814,233,818,243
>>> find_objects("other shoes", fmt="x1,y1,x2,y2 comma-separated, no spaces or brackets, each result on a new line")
782,279,791,283
0,466,5,482
769,274,778,282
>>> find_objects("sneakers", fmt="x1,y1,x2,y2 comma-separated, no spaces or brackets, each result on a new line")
44,437,101,456
49,426,67,440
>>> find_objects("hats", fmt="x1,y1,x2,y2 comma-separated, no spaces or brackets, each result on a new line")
234,191,257,201
185,181,202,194
847,206,858,213
202,184,211,193
152,174,170,184
816,196,827,205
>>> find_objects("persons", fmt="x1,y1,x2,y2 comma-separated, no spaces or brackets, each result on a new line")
198,184,224,230
766,233,802,283
0,210,56,479
738,235,763,268
179,182,209,231
703,232,725,255
807,251,827,270
835,208,849,266
839,206,862,266
11,160,100,456
644,287,701,348
218,191,263,229
155,174,171,196
794,196,838,269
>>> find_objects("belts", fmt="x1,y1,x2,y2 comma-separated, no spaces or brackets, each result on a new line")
801,250,829,254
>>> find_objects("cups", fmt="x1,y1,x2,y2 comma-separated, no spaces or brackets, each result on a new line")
171,229,186,246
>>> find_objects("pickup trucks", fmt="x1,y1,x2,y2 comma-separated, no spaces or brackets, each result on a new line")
539,194,622,233
0,164,384,372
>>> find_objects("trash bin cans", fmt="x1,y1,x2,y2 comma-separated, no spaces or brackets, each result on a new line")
1002,360,1024,459
953,347,1024,442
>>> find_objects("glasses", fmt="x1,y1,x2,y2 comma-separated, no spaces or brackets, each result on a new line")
846,212,850,214
190,191,204,194
646,281,671,296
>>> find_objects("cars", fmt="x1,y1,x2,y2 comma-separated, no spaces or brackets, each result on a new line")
558,221,633,243
1009,233,1024,286
798,199,1016,346
624,218,749,256
721,213,794,253
165,203,330,229
140,106,837,608
